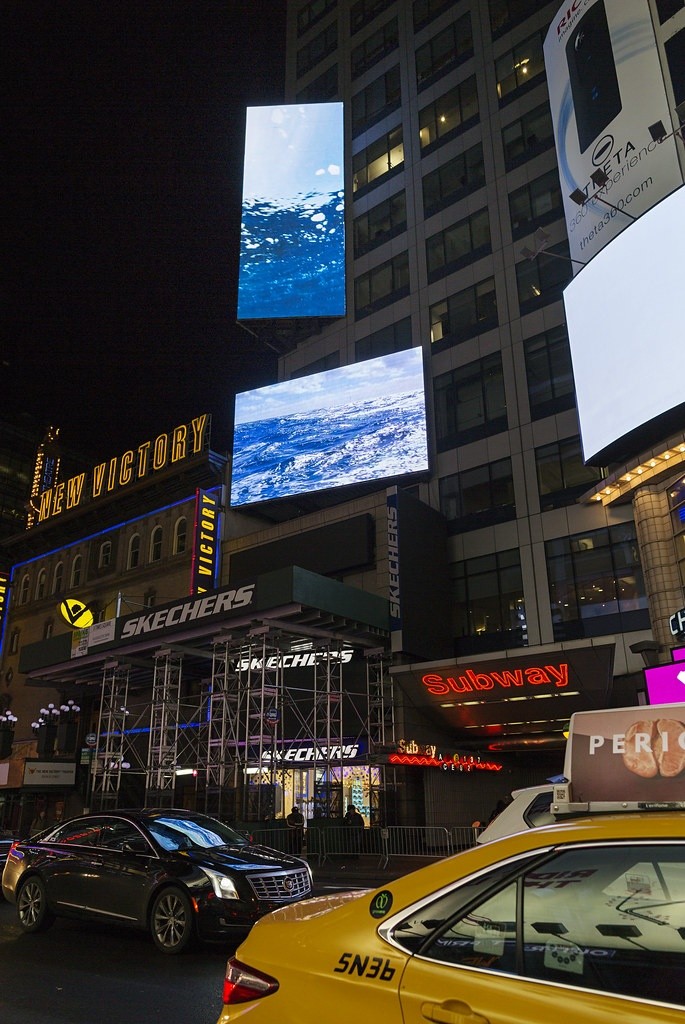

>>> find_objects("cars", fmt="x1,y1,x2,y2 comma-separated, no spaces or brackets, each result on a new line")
1,807,315,955
0,834,22,866
219,704,685,1024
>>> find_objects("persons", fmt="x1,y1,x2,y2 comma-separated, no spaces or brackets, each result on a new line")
286,805,305,858
488,800,508,824
29,810,50,837
344,804,366,861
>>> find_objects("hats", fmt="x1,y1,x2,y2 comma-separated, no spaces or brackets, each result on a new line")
292,807,300,810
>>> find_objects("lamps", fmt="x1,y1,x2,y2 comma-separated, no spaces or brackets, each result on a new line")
569,168,637,221
597,925,650,952
531,921,583,949
521,227,588,266
649,102,685,144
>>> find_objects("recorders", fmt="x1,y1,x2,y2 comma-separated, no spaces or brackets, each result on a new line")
565,0,624,154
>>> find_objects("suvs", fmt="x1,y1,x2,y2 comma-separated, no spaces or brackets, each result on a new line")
443,785,685,953
